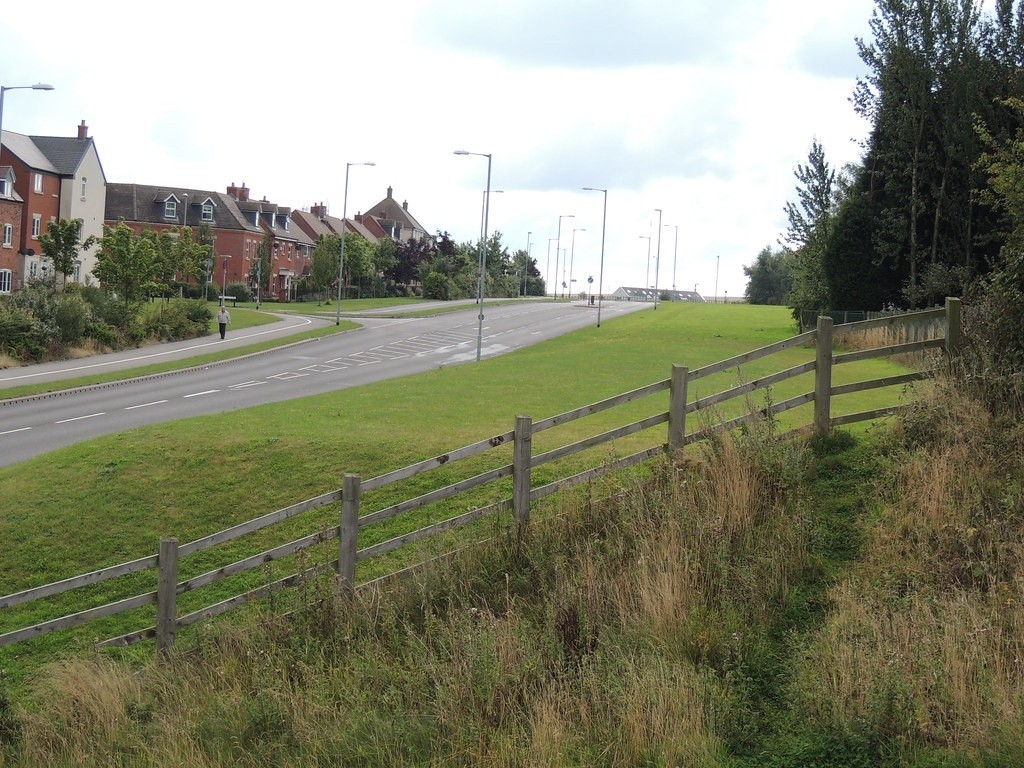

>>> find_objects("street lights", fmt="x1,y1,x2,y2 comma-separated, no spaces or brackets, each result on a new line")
477,190,504,303
454,150,491,360
336,162,375,326
525,232,533,297
639,236,650,299
569,228,586,298
665,225,677,300
555,215,576,299
559,247,567,298
546,239,557,291
582,187,608,328
653,209,662,309
715,256,719,300
0,84,55,136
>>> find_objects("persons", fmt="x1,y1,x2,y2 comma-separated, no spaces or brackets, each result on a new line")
216,306,231,340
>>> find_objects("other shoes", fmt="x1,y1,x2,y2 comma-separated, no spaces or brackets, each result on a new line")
220,338,224,339
